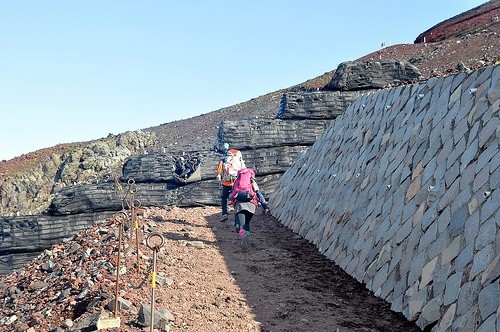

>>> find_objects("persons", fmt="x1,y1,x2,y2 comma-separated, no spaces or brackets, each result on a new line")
229,168,269,237
216,148,247,222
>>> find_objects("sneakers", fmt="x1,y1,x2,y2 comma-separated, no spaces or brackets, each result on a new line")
219,214,228,222
241,235,250,252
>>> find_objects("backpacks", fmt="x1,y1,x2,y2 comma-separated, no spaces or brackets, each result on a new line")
223,148,246,181
232,168,256,202
